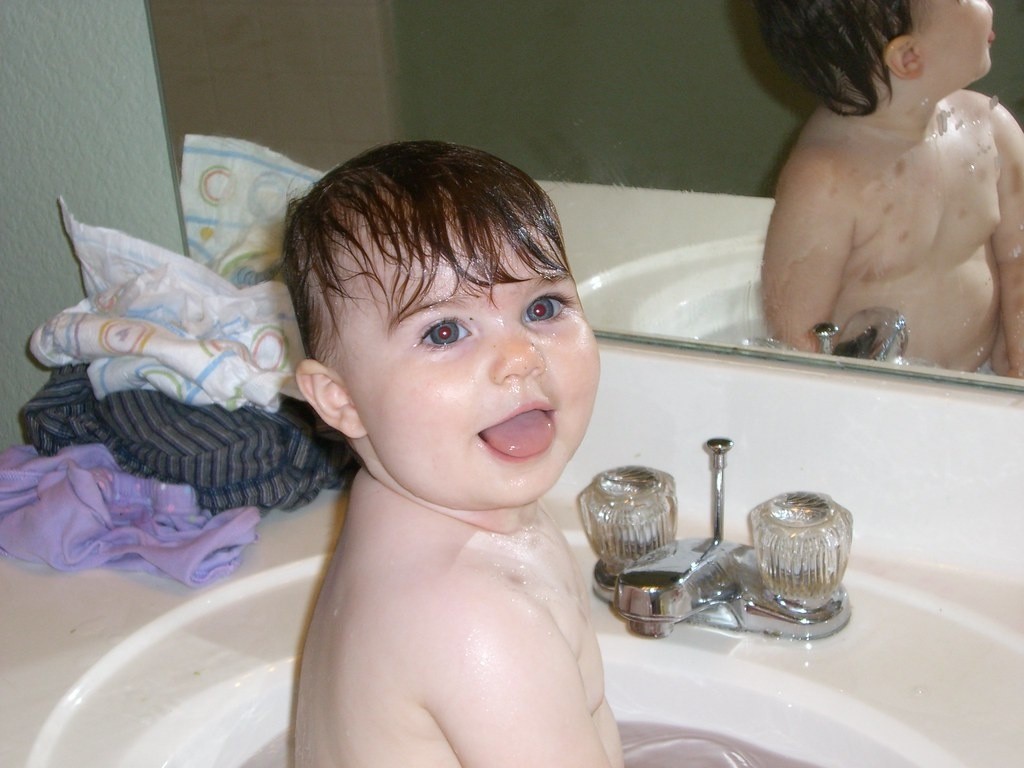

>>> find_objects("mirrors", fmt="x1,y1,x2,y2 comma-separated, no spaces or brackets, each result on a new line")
142,0,1024,395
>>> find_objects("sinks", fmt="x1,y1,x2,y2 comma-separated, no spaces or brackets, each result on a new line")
22,528,1024,768
572,222,772,350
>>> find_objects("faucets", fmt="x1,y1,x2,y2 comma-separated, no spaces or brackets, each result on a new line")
832,305,909,365
609,534,737,637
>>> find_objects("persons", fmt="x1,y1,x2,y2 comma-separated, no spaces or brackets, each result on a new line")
283,141,629,768
752,0,1024,379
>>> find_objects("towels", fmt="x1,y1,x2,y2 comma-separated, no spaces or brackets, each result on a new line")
179,131,329,292
0,197,360,590
